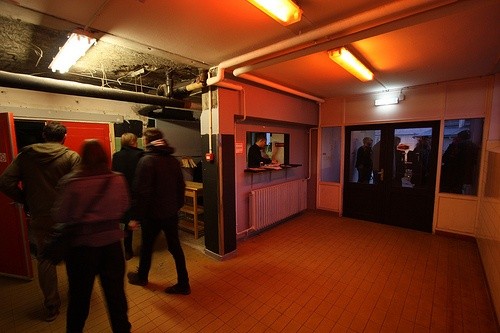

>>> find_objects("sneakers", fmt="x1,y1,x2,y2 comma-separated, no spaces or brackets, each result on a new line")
127,270,148,285
166,284,191,294
44,302,61,321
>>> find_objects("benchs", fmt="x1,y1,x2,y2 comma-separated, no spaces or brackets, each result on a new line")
177,191,204,240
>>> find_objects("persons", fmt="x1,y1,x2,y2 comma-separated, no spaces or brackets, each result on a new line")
355,137,372,184
440,128,472,194
0,122,83,322
51,139,132,333
111,133,145,260
371,137,405,187
128,127,191,295
193,159,205,220
409,136,432,188
248,136,278,168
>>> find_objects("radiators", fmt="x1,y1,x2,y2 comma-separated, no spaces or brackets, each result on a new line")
249,178,308,231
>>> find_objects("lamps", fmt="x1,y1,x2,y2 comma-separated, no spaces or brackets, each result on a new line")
246,0,304,27
48,30,97,74
328,47,375,83
